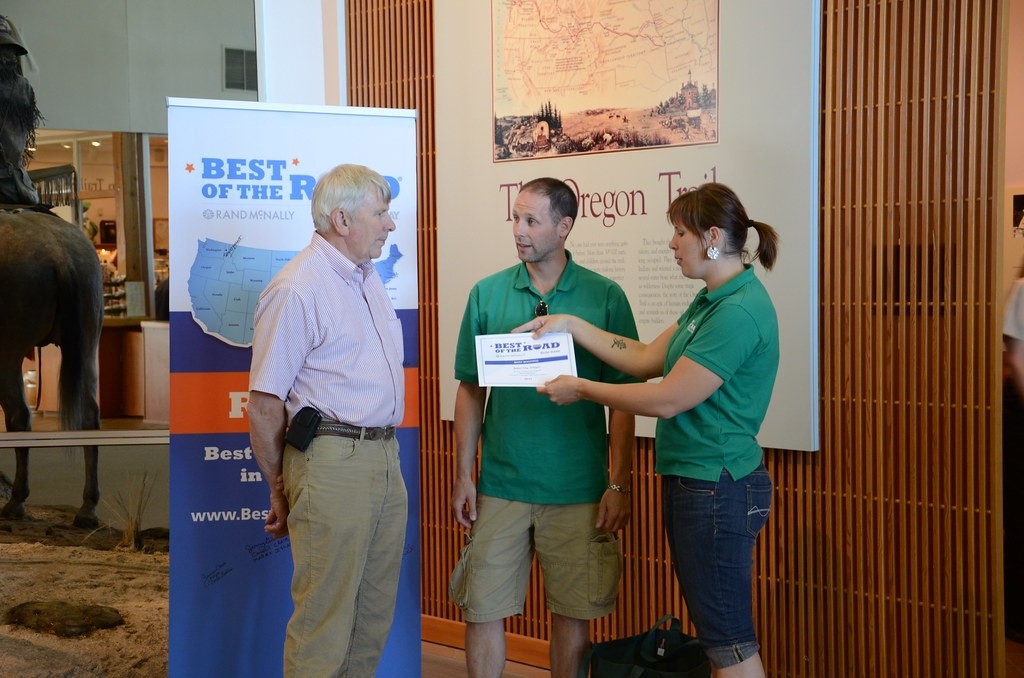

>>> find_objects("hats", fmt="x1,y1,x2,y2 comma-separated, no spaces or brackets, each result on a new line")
0,14,39,74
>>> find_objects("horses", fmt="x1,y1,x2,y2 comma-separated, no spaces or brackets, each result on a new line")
0,208,105,529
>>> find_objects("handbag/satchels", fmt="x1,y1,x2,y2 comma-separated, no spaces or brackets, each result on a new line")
577,614,711,678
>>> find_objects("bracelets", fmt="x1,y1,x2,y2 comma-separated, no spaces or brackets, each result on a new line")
605,483,633,496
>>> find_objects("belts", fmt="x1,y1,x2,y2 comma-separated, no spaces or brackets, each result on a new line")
316,422,397,442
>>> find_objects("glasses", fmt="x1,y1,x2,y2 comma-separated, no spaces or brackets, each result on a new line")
535,300,549,316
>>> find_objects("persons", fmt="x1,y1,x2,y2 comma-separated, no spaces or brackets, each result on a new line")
242,162,419,677
1000,272,1024,403
450,176,651,678
510,180,783,678
